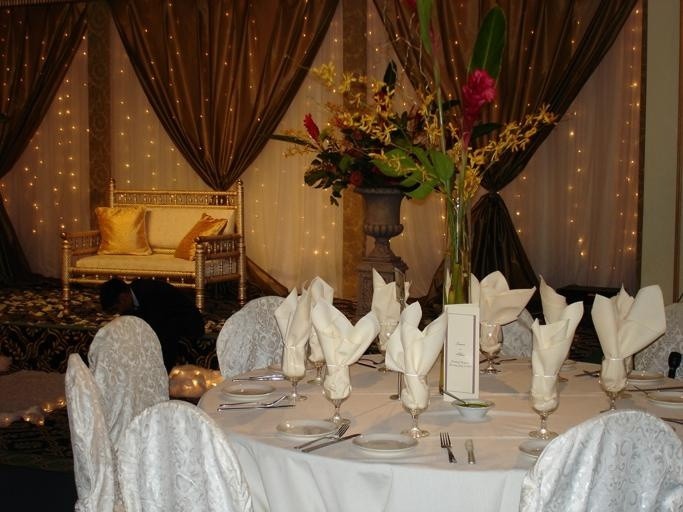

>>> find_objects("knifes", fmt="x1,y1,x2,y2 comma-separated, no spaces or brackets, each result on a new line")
302,434,361,453
217,404,296,411
465,439,475,464
628,385,683,392
231,378,288,382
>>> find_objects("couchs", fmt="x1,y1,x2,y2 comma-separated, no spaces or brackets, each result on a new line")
60,177,247,313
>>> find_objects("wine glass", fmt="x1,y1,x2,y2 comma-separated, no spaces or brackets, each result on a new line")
282,321,430,424
480,321,633,440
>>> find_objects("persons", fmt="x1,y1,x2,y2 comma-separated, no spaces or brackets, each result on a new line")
99,275,204,375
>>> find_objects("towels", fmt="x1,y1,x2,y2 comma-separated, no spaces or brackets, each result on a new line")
531,275,585,410
590,283,667,390
306,275,333,361
311,296,381,398
468,270,535,352
384,301,447,408
274,280,314,377
372,267,412,350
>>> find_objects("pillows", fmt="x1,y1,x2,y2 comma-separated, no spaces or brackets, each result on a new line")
174,213,228,261
95,206,152,256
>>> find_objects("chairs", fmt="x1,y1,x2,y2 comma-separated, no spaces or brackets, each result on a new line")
518,410,683,512
117,401,253,512
66,353,123,511
87,315,170,438
501,306,534,357
216,295,318,379
634,301,683,381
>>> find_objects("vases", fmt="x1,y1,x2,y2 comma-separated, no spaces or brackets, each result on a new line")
438,197,471,395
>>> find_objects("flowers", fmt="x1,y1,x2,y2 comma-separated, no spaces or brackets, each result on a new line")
270,0,561,394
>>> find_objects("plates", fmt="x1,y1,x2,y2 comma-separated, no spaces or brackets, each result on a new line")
518,440,548,458
272,364,314,373
353,432,418,453
627,370,667,381
647,391,683,408
455,415,491,423
223,385,276,399
276,419,336,437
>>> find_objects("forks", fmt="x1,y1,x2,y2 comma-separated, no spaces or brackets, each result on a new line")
295,425,350,449
221,396,287,406
440,432,456,463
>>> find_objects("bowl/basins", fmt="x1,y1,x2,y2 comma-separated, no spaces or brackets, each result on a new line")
452,399,494,418
